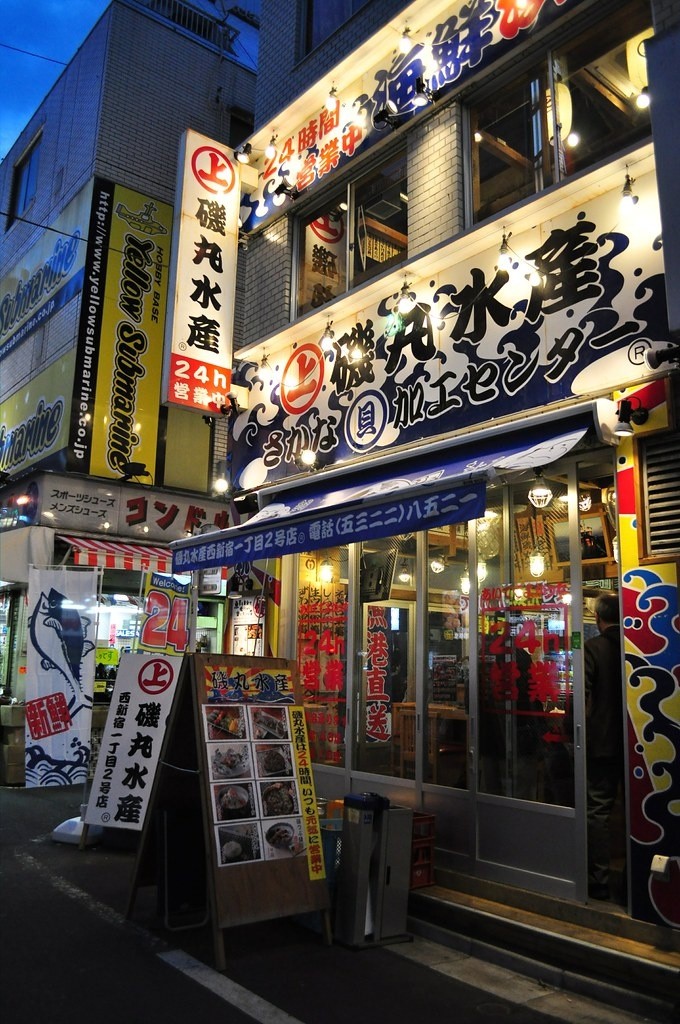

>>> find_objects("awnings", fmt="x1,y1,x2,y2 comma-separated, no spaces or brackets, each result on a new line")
166,428,589,574
56,536,191,574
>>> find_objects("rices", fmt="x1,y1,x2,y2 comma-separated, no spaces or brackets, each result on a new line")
222,841,242,858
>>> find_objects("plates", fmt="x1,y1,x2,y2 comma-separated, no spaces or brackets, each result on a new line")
207,711,245,737
264,789,294,814
253,711,287,738
211,753,248,776
256,748,291,776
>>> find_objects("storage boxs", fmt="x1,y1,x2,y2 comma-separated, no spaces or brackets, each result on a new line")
301,799,437,935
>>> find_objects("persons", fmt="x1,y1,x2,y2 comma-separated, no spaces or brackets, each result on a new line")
391,661,407,702
583,595,628,907
464,604,544,801
458,656,469,683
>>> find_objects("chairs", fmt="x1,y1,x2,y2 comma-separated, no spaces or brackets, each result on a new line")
398,709,469,786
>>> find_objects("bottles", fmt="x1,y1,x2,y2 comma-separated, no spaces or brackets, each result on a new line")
456,684,465,708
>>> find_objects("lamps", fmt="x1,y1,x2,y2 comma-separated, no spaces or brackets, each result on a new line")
415,76,440,105
221,391,240,416
328,203,348,222
275,183,300,202
373,109,400,132
399,465,592,595
637,85,651,108
236,143,265,164
613,396,648,437
644,344,680,371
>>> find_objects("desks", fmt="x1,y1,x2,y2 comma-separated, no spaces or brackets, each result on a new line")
391,702,467,778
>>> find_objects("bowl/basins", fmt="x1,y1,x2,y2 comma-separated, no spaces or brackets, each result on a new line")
222,841,242,860
266,822,294,848
218,787,248,812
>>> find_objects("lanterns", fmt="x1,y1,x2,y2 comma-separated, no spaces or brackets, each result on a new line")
546,82,573,146
626,28,654,93
